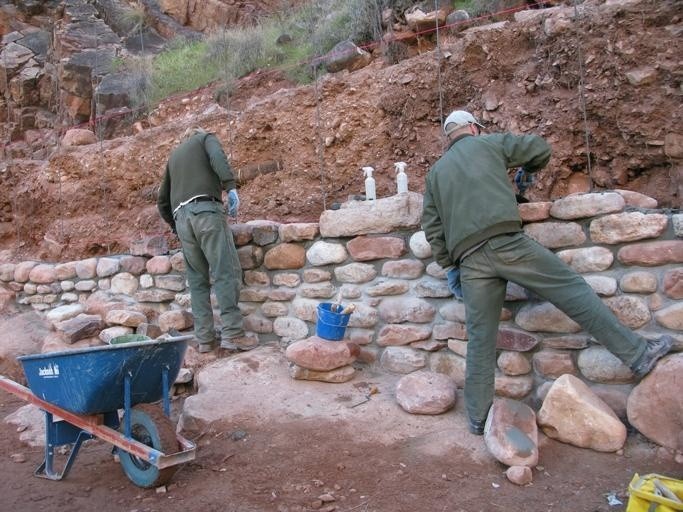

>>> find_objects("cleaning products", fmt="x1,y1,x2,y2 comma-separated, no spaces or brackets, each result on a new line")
362,167,376,199
394,162,408,193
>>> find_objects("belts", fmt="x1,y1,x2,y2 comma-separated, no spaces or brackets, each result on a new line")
189,195,224,205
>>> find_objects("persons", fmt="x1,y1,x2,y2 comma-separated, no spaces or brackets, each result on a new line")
157,124,258,354
420,111,675,433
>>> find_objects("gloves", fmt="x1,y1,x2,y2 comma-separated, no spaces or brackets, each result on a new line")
226,189,241,218
514,169,536,192
443,264,463,299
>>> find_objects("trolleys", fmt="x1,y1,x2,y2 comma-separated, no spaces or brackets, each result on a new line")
0,331,202,489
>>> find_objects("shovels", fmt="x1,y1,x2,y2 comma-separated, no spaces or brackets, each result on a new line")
515,174,529,204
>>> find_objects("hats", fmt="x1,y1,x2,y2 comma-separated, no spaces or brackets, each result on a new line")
443,110,486,141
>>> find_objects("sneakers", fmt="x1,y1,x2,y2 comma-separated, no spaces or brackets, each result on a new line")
469,416,484,435
199,335,258,354
629,334,672,378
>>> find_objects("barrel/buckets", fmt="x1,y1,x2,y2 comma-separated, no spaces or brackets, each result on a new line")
316,304,350,341
